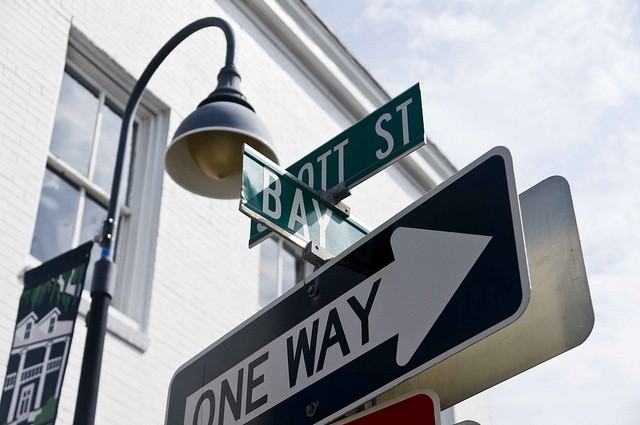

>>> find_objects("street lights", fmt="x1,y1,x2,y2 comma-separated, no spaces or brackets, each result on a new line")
72,15,281,425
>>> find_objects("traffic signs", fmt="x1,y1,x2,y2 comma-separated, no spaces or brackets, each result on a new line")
238,138,372,275
248,71,427,252
162,143,534,425
